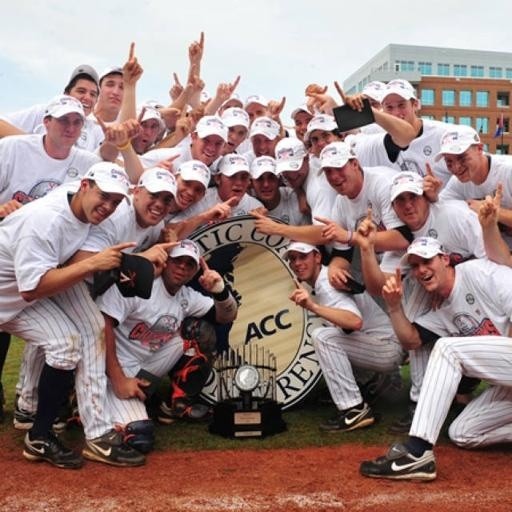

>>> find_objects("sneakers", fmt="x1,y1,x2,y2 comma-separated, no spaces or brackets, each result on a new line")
319,402,375,433
82,431,146,466
362,375,392,401
389,402,417,435
23,433,83,468
360,443,437,481
158,400,212,424
13,393,81,429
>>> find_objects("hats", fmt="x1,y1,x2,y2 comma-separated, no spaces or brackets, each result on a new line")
389,172,425,202
166,239,200,274
83,161,131,206
362,82,387,103
138,90,358,203
400,237,446,266
43,96,86,122
280,242,320,258
113,252,153,299
435,125,482,162
380,78,418,104
100,67,123,81
65,64,100,92
